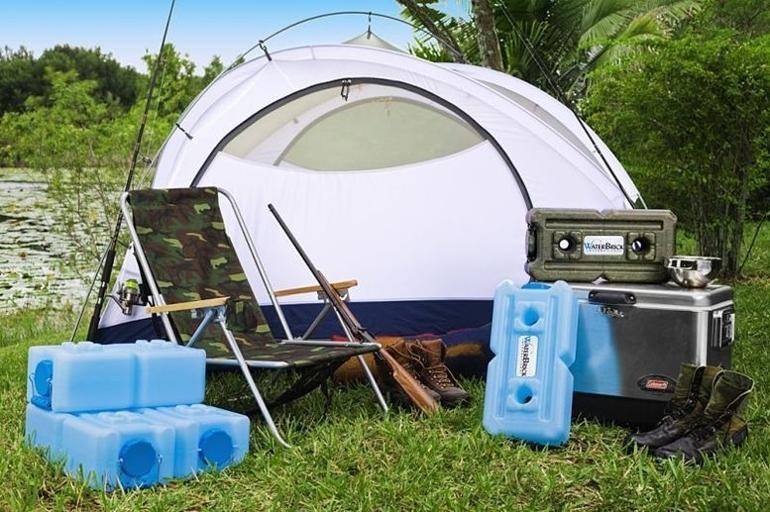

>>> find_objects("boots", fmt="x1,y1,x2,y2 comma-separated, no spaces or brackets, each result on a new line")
411,338,472,409
373,340,441,407
623,361,755,469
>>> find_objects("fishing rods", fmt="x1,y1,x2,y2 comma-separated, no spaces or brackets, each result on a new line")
88,0,176,341
494,0,639,209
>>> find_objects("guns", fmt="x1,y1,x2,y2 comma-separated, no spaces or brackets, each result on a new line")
269,204,442,416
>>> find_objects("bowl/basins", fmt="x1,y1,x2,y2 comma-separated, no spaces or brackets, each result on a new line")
663,256,722,288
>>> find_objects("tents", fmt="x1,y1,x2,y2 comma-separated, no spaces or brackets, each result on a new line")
92,10,650,383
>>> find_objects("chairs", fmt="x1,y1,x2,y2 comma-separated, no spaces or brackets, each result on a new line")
121,185,394,450
